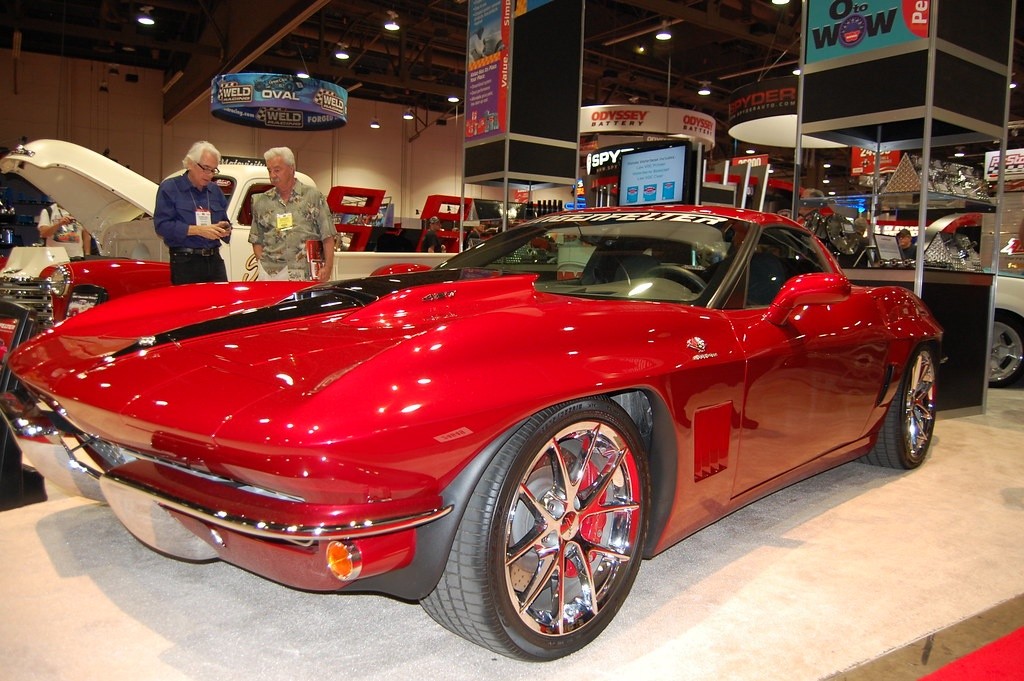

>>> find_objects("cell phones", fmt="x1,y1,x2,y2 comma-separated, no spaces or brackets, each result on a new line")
218,223,232,232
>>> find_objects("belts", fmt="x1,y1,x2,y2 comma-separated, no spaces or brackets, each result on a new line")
168,247,219,256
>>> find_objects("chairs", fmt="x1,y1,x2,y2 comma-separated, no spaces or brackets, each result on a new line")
575,253,666,286
691,253,784,306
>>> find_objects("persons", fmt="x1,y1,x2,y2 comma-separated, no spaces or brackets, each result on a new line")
36,203,91,260
421,217,446,253
463,221,485,254
248,147,336,283
896,229,917,259
153,140,232,285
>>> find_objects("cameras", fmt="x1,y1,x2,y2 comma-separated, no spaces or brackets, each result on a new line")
69,217,77,223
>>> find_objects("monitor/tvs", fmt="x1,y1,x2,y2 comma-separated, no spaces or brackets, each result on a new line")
617,140,693,205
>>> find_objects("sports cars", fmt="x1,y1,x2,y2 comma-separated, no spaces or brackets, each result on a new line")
0,202,947,663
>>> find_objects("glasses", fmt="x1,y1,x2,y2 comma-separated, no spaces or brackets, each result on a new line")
197,162,220,175
899,236,910,240
69,218,77,223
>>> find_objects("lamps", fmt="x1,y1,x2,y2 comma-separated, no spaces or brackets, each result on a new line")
697,80,712,96
384,9,400,30
333,39,349,60
137,4,155,25
655,20,673,40
369,101,381,128
139,9,140,10
403,105,413,120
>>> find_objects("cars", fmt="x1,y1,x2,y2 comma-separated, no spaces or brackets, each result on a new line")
0,139,1024,389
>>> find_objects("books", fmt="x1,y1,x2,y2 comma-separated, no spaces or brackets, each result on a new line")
305,239,326,279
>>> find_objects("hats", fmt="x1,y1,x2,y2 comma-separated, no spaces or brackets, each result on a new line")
428,216,440,223
